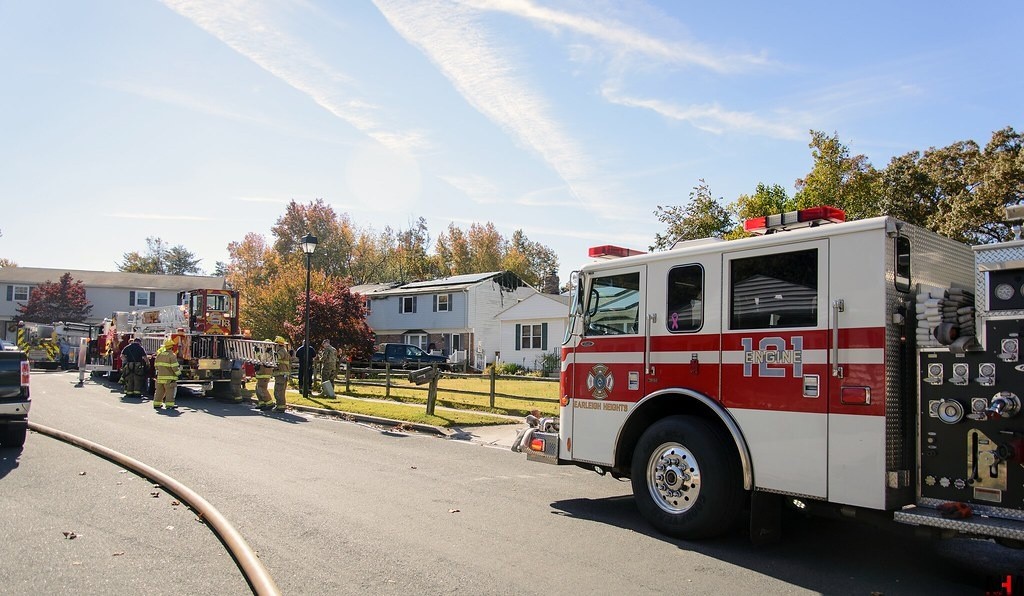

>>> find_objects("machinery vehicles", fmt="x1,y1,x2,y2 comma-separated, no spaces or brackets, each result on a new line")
86,289,251,394
17,321,61,370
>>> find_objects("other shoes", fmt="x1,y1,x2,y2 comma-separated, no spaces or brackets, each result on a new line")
127,393,132,397
255,406,262,409
271,407,284,412
261,406,272,410
167,405,178,409
134,394,141,397
154,405,163,409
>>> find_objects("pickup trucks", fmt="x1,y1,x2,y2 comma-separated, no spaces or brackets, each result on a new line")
339,343,450,375
0,339,32,448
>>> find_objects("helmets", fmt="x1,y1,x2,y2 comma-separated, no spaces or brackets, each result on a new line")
164,339,174,346
264,338,272,342
128,338,134,342
275,337,285,343
134,338,141,342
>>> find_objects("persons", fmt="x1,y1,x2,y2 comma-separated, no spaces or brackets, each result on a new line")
284,342,344,389
120,339,134,394
318,339,337,396
254,339,275,411
60,337,70,372
153,339,184,408
120,337,150,397
272,336,289,413
296,339,316,394
229,345,258,404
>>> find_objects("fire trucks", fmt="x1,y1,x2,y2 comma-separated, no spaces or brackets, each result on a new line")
511,205,1024,551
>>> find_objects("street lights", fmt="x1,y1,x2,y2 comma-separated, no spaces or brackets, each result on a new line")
301,233,319,398
